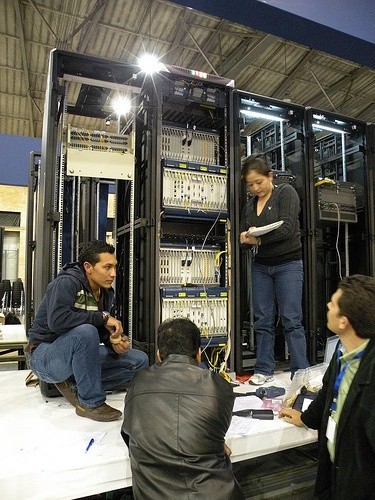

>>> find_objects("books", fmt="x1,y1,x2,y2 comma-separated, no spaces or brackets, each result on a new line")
246,220,284,236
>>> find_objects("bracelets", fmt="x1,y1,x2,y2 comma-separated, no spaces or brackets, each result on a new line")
253,237,259,254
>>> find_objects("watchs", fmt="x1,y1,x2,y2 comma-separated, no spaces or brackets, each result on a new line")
103,311,110,324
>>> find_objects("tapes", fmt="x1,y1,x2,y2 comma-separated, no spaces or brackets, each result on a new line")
110,334,122,344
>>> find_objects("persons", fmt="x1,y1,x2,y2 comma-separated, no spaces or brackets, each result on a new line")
240,155,308,386
24,240,149,422
121,319,246,500
278,274,375,500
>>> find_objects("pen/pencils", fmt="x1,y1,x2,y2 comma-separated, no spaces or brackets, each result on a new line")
85,438,94,453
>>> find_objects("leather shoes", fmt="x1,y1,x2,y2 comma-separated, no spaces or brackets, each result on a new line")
54,381,77,407
76,402,122,422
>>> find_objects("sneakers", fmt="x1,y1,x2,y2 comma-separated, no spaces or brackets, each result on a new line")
249,373,274,385
